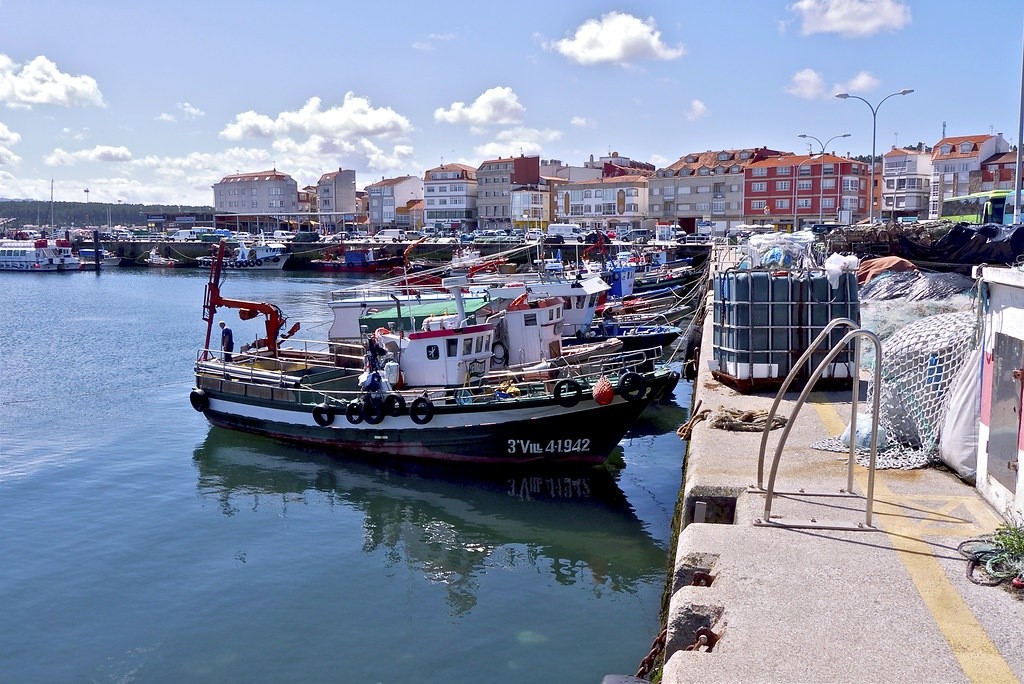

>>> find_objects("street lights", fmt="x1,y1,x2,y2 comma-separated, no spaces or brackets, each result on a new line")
798,132,851,225
836,87,914,224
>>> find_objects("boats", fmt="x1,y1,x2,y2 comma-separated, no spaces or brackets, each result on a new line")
0,194,711,468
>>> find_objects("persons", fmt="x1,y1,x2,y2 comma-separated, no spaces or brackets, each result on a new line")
219,321,234,362
0,228,30,241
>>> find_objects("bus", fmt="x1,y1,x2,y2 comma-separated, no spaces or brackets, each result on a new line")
941,188,1017,228
1004,190,1024,228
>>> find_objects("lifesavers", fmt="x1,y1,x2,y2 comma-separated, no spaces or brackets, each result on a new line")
505,283,524,287
509,293,527,306
